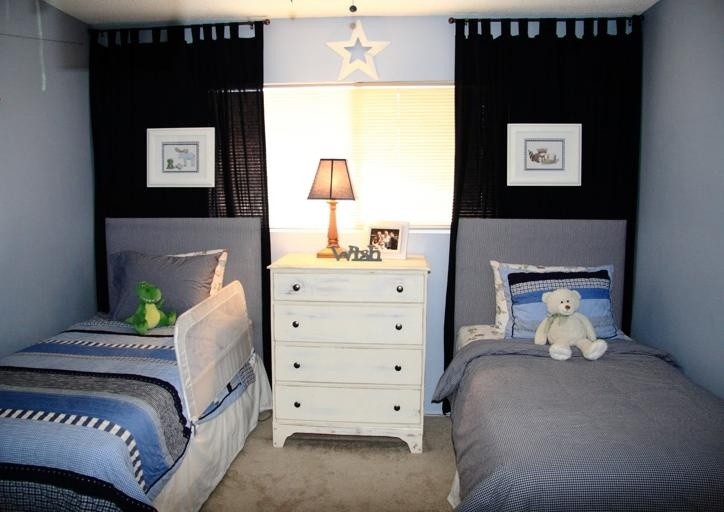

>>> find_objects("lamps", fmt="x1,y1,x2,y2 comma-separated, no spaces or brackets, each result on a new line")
308,159,355,258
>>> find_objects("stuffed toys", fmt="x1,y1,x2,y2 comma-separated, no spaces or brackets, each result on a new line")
534,288,608,360
124,280,177,334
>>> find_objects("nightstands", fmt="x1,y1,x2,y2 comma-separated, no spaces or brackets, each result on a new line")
266,255,431,453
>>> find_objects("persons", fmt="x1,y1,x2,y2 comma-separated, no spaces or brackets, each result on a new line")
372,230,398,249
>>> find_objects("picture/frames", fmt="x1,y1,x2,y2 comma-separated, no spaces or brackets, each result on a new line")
507,124,582,186
147,127,215,188
363,221,410,260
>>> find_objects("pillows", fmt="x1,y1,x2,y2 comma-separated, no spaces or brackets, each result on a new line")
107,249,228,321
490,259,633,341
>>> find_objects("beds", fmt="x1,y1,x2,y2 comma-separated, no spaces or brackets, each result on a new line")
432,218,723,511
1,217,272,512
